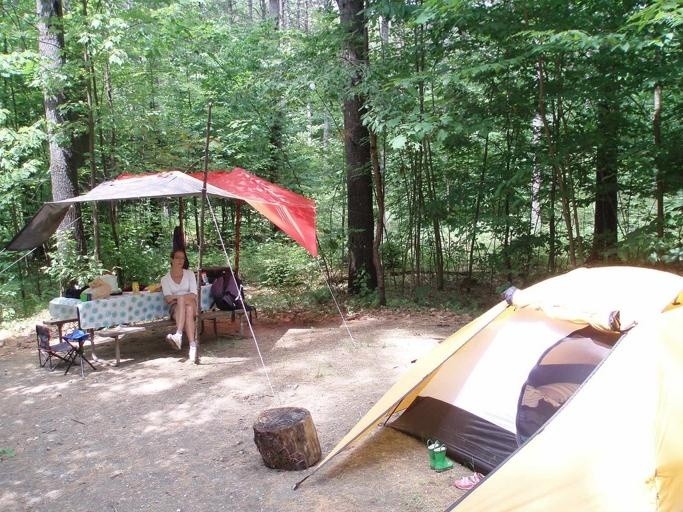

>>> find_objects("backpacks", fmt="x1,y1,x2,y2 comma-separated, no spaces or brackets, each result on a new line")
210,271,246,311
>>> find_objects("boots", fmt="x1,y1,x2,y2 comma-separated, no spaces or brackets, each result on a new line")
428,443,439,469
433,447,453,473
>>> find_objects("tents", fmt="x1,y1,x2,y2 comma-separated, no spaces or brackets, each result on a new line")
291,266,683,512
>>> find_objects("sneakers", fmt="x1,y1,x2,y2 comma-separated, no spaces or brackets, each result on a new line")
188,347,199,364
165,333,182,352
454,471,486,490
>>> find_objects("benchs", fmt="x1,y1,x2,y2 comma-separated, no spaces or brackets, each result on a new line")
43,319,78,344
94,306,258,367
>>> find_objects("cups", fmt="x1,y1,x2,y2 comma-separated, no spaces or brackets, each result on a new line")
132,281,139,294
80,293,91,302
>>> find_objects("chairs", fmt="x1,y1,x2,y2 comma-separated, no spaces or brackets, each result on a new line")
37,326,78,372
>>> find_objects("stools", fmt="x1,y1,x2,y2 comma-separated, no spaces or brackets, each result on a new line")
61,329,97,379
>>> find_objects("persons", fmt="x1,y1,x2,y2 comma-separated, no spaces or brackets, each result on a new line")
160,249,197,362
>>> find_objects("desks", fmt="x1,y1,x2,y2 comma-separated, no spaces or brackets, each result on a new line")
49,284,217,360
